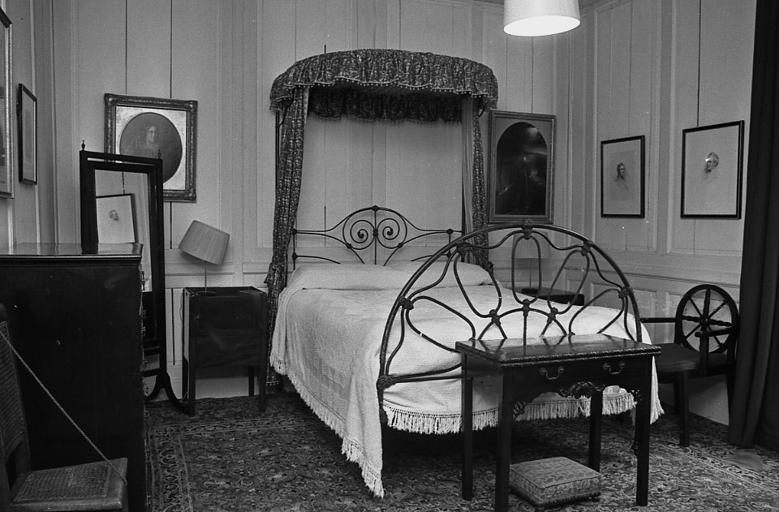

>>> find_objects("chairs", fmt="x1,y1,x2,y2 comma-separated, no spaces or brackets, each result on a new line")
0,322,129,512
642,284,740,447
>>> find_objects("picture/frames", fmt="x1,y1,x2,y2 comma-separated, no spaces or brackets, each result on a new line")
486,108,557,226
96,192,138,243
18,82,38,186
600,134,645,219
680,120,744,220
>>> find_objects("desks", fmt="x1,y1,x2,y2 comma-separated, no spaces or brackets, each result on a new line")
455,331,660,512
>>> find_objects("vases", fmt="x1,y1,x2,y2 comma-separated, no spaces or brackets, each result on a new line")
103,92,198,204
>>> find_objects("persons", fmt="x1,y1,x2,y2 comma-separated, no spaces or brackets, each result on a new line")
134,123,165,158
617,163,625,180
706,151,719,172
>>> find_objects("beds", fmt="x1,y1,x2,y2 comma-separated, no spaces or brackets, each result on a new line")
265,206,650,490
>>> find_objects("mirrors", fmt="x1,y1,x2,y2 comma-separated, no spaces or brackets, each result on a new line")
79,140,191,417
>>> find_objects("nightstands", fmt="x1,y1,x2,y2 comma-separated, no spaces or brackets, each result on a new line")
181,286,268,412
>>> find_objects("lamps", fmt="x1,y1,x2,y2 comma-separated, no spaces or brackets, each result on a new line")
502,1,582,36
179,220,231,296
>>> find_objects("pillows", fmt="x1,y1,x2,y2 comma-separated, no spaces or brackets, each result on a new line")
290,259,498,290
